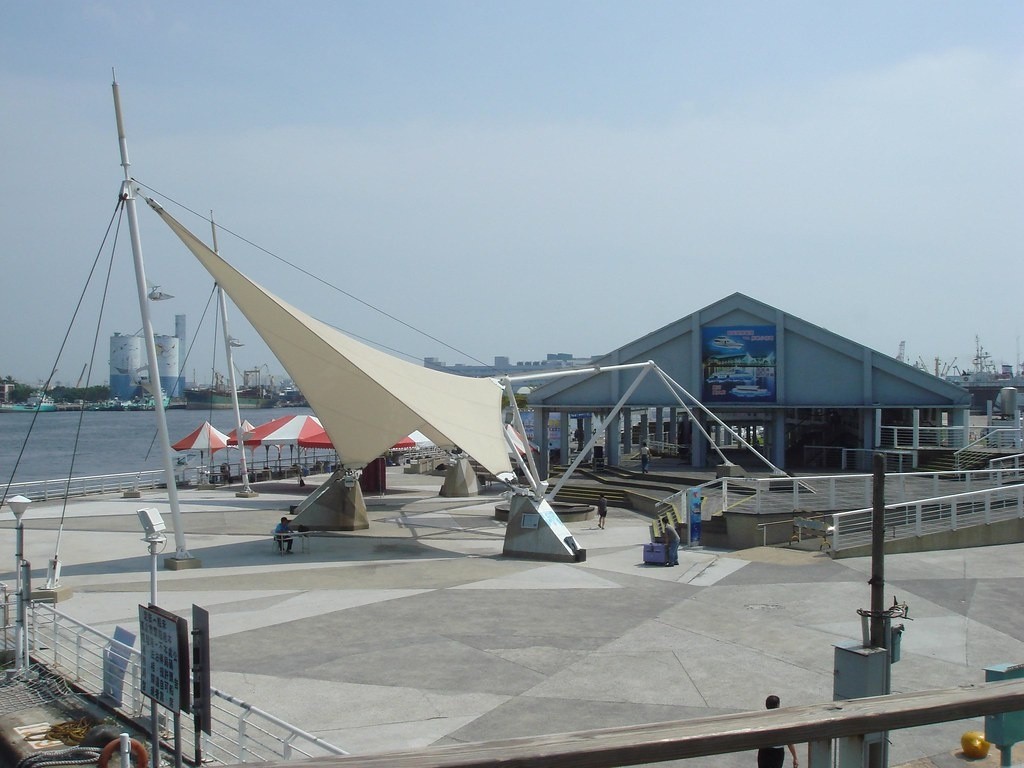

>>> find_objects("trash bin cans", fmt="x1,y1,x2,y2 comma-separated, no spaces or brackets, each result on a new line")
891,623,905,664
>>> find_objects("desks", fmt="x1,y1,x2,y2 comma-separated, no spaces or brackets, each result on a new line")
206,473,224,484
249,469,262,482
274,530,321,556
305,463,314,475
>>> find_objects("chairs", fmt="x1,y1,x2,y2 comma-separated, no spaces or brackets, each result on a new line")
271,529,293,555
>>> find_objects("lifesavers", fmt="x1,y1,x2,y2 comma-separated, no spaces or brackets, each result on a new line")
99,737,146,768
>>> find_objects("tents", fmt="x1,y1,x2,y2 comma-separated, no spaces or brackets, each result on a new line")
504,424,538,480
227,414,440,486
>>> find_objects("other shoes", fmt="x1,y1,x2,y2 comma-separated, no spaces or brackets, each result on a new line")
602,527,604,529
280,548,284,552
665,564,674,567
286,550,293,554
598,524,601,528
674,563,679,565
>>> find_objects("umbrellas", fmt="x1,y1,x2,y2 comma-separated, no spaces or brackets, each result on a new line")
170,415,256,484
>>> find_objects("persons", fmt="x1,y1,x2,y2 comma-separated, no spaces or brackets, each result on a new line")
640,442,651,473
691,491,701,542
660,517,680,567
757,695,799,768
598,493,608,529
274,516,295,553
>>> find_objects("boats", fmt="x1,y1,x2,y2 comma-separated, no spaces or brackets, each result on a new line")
128,388,169,411
0,390,58,412
182,362,276,409
